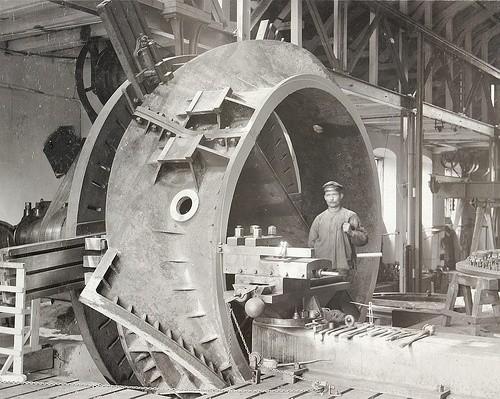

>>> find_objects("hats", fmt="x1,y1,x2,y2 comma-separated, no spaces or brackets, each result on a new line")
323,181,343,193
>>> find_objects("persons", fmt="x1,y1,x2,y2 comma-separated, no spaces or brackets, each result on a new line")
307,182,370,303
